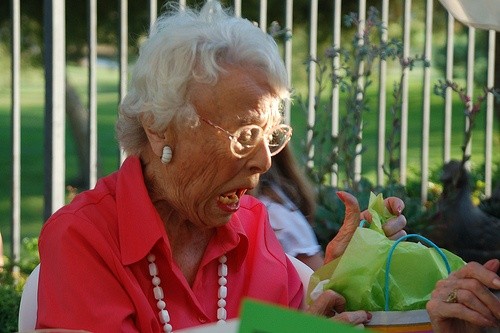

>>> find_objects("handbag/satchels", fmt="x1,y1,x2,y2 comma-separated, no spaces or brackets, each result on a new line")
306,219,467,333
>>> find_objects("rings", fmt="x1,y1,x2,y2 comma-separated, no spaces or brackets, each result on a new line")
444,290,459,303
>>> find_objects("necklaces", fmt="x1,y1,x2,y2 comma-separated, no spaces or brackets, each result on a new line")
148,249,227,333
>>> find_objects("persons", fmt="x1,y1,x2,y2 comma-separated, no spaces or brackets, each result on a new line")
248,118,324,272
35,0,407,333
424,259,500,333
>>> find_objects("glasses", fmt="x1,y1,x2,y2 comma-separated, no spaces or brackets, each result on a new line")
198,113,293,158
